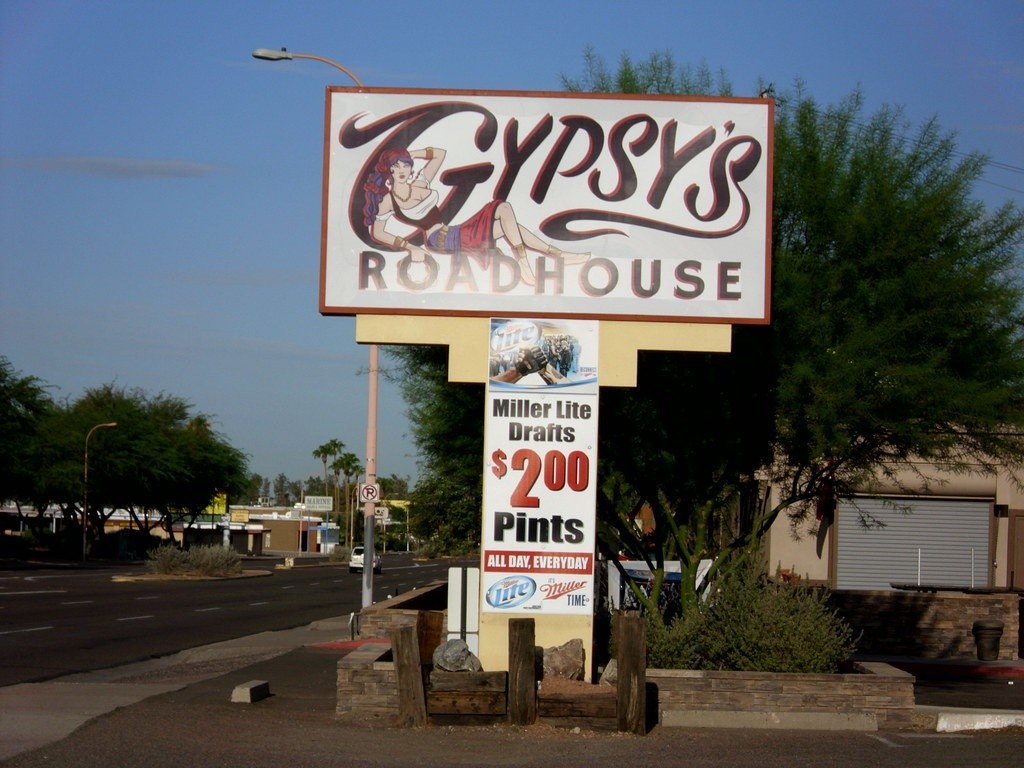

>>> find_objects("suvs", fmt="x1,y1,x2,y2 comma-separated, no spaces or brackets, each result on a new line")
348,546,383,574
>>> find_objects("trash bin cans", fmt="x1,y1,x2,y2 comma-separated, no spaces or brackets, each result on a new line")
971,620,1005,661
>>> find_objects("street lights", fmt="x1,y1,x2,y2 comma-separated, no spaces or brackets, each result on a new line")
83,422,117,556
252,47,379,607
